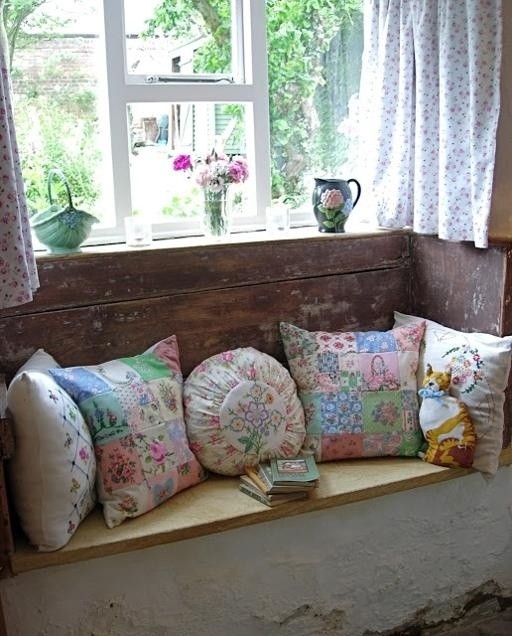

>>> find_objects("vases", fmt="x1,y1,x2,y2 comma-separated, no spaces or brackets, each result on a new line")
197,184,231,239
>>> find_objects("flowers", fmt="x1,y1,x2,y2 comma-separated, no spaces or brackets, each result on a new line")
172,147,249,193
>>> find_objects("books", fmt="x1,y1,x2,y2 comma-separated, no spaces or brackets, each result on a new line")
236,449,320,508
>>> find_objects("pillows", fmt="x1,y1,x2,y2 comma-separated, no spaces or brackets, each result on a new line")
6,347,95,552
48,334,208,529
279,320,425,461
182,347,307,475
391,312,512,476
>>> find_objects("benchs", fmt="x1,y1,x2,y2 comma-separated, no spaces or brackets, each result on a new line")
0,230,512,579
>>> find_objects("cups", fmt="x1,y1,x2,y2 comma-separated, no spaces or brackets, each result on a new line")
266,206,290,237
125,216,151,248
